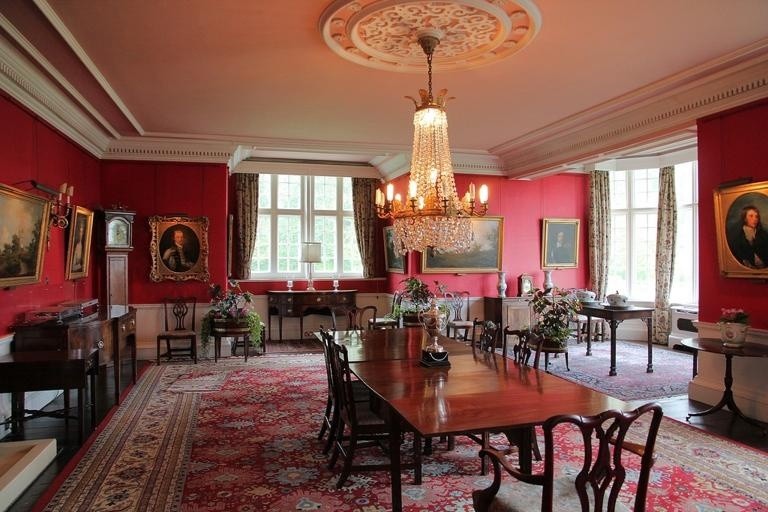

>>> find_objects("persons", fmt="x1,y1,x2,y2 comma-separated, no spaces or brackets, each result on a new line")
71,216,85,272
162,227,198,273
546,224,574,263
725,206,768,269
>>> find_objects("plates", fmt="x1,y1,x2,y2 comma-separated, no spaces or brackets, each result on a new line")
600,302,634,310
579,301,600,306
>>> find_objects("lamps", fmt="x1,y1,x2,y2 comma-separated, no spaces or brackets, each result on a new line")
375,32,489,257
303,241,321,292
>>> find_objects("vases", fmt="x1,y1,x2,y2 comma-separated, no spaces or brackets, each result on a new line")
214,318,249,328
717,320,752,348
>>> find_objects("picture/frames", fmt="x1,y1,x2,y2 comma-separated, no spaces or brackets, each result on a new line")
65,204,94,279
420,216,505,276
713,181,768,279
382,226,406,274
541,217,580,269
0,183,49,287
149,214,211,282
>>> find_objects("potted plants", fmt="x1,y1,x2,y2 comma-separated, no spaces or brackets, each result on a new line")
525,289,578,350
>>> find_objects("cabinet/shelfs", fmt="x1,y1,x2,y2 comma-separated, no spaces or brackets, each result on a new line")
503,298,568,349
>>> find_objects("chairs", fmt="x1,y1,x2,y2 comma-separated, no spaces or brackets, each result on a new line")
232,322,267,356
447,325,547,476
157,297,198,364
316,323,375,454
328,339,423,488
471,401,663,510
326,289,498,352
559,290,605,344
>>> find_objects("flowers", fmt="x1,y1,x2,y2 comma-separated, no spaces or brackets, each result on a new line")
200,280,265,362
719,305,747,324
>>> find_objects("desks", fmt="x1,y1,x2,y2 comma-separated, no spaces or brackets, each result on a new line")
11,320,117,430
315,325,480,363
521,340,571,373
267,289,358,343
214,328,250,363
680,338,768,428
115,310,138,404
572,302,656,376
348,352,637,511
0,348,99,445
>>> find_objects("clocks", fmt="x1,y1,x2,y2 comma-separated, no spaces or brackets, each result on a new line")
106,211,135,251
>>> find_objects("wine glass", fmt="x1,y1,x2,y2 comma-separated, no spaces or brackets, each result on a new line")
287,280,294,291
333,279,339,291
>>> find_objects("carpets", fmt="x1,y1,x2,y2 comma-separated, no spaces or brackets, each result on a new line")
30,350,768,512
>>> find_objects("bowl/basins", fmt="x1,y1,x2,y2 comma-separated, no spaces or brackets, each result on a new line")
606,295,628,305
575,292,597,301
